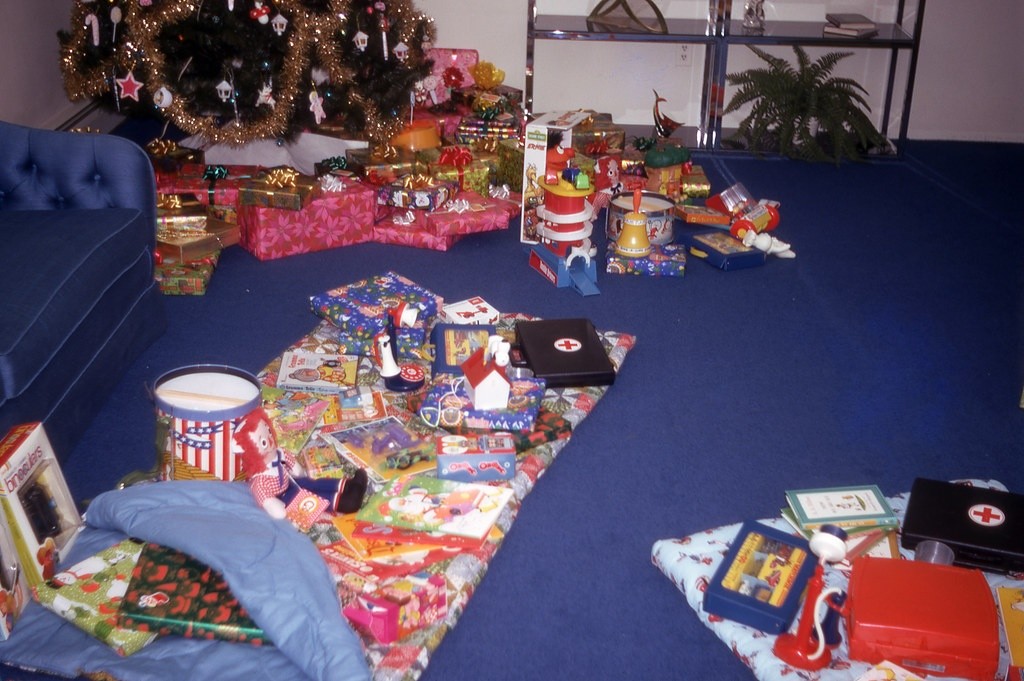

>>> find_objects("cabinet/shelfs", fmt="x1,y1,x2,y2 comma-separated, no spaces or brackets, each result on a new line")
712,0,926,165
525,0,725,155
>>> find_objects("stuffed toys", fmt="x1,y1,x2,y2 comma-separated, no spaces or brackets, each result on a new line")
232,409,368,520
547,131,575,171
590,155,628,222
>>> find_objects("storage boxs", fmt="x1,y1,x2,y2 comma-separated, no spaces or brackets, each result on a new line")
423,373,546,431
702,518,817,635
436,432,518,483
0,421,83,596
154,48,784,288
430,322,497,376
310,271,444,339
847,554,999,681
900,477,1024,574
508,317,617,389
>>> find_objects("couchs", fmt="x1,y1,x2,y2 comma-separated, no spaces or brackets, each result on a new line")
0,120,157,448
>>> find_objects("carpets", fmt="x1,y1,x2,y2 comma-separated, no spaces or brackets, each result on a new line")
649,478,1024,681
0,310,636,681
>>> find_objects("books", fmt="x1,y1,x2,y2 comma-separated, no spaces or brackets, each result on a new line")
261,352,572,561
824,13,877,36
781,485,898,570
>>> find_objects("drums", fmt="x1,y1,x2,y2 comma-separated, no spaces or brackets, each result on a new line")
152,363,264,484
606,191,676,247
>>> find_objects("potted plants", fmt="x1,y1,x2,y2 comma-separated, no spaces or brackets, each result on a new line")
722,43,888,168
